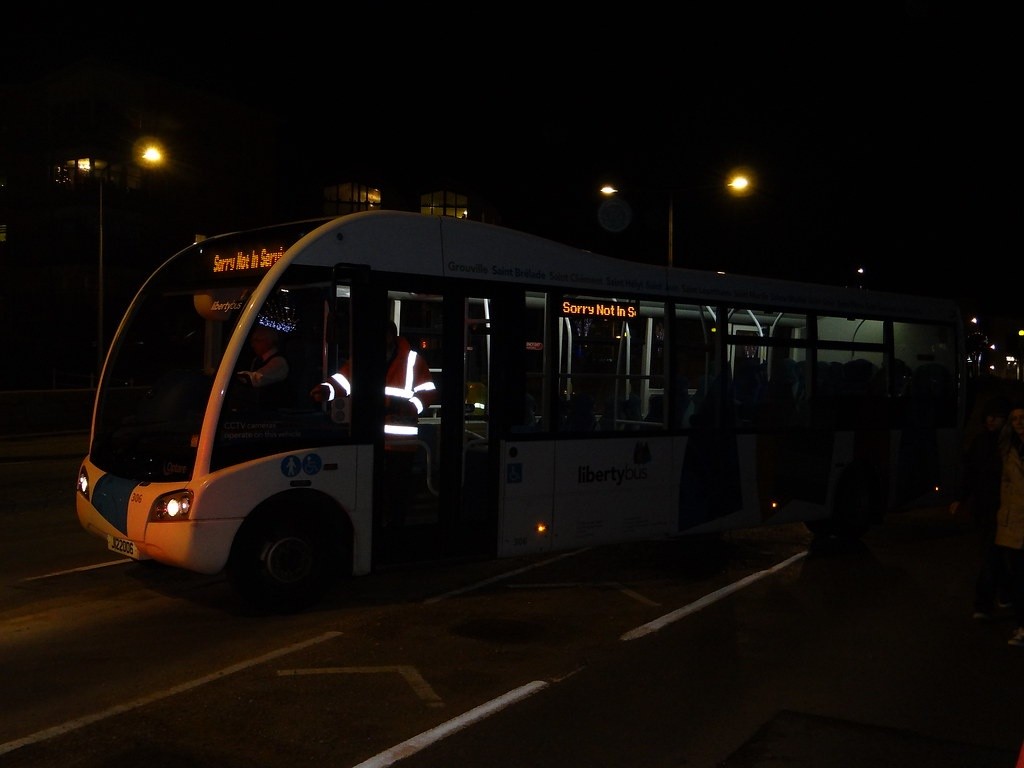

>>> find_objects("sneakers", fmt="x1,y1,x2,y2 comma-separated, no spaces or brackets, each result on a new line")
994,592,1012,608
1007,626,1023,646
974,607,992,623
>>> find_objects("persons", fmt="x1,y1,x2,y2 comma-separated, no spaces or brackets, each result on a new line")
308,319,440,564
974,398,1024,653
204,323,288,416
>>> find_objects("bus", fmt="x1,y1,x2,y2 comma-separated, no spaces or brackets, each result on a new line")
76,209,968,616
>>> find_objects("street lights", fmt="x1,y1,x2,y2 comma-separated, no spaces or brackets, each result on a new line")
95,135,173,391
666,166,758,265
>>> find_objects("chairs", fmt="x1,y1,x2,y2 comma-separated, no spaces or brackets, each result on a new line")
600,399,642,432
642,404,682,430
546,405,596,433
